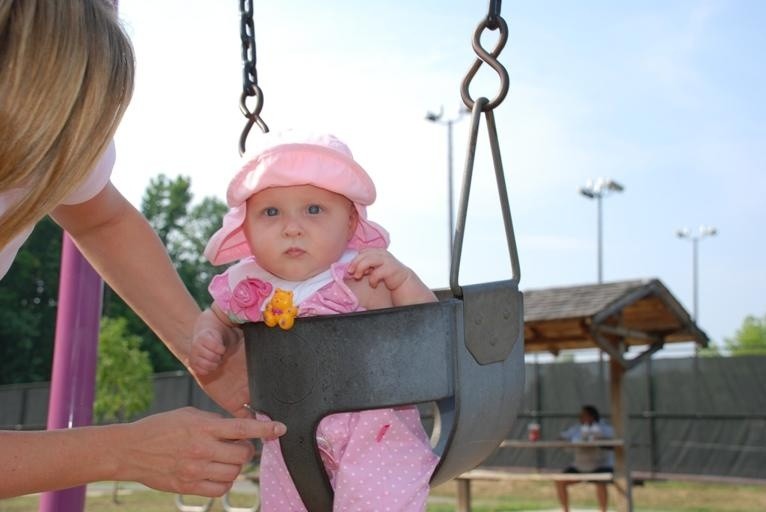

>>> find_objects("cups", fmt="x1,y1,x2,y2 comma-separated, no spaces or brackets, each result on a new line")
528,424,541,443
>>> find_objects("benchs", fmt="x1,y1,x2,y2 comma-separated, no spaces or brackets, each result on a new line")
454,469,614,510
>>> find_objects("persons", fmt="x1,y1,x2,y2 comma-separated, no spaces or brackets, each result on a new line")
188,133,440,510
556,405,616,511
1,1,287,501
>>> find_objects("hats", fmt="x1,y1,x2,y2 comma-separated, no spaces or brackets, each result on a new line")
205,135,391,267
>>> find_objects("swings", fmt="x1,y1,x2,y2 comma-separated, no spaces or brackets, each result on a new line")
239,0,525,512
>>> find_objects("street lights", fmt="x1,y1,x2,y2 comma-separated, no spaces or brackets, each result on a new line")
577,178,624,285
425,100,472,263
676,227,717,327
